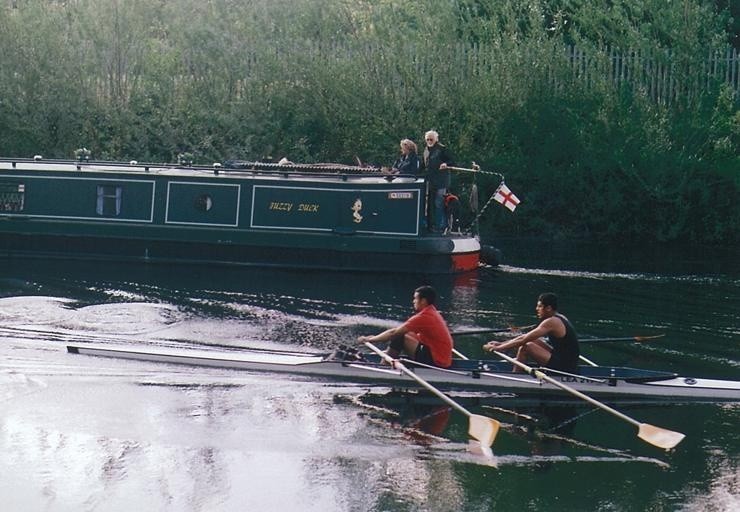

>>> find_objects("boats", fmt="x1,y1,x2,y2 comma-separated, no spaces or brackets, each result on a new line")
0,147,505,276
63,333,739,406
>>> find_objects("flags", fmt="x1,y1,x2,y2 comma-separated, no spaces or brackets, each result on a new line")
491,182,522,214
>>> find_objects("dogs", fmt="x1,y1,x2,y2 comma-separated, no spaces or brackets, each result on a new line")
441,189,464,237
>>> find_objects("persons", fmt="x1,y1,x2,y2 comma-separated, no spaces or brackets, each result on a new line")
484,291,582,376
387,138,421,177
423,130,455,235
355,283,453,368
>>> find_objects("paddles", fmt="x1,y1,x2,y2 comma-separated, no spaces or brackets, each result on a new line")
367,341,501,448
450,323,533,340
577,334,668,346
491,350,686,450
